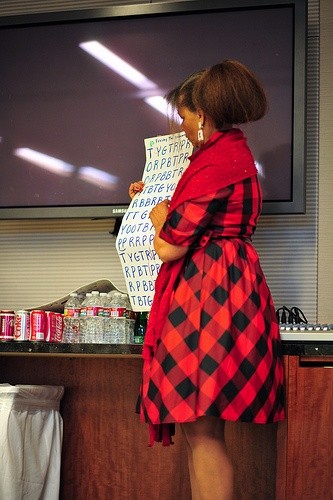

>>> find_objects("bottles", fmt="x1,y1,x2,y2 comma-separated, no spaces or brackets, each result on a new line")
61,291,147,344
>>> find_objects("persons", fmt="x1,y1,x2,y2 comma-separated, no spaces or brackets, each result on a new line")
129,58,287,500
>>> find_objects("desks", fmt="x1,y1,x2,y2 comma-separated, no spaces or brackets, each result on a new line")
0,342,333,500
0,383,64,500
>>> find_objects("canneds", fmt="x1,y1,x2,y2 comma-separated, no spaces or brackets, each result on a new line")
0,309,66,342
